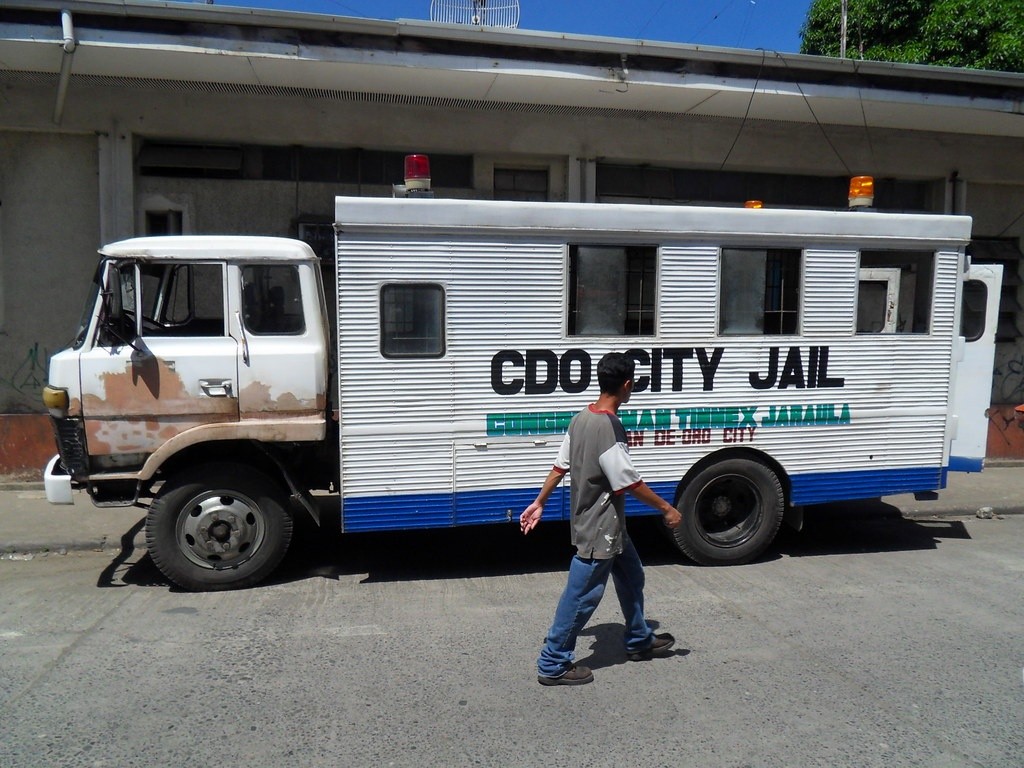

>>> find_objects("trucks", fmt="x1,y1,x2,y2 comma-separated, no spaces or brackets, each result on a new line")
38,152,1006,595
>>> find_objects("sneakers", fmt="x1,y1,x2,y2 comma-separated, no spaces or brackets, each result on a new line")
628,632,674,660
538,665,594,685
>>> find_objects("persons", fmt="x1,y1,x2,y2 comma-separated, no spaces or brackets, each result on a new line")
520,352,682,686
